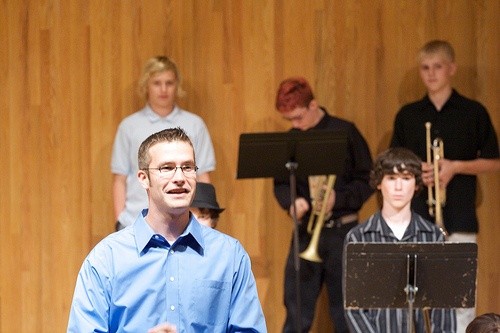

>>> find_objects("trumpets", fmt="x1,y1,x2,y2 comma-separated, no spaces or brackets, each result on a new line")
299,175,336,263
425,120,447,239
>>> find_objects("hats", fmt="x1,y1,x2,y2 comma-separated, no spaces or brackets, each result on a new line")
190,182,226,213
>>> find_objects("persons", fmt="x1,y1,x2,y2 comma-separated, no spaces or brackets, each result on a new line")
389,40,500,333
110,56,225,232
273,77,377,333
466,313,500,333
342,149,457,333
66,127,268,333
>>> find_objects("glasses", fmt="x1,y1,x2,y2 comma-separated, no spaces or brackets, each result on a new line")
140,163,199,174
